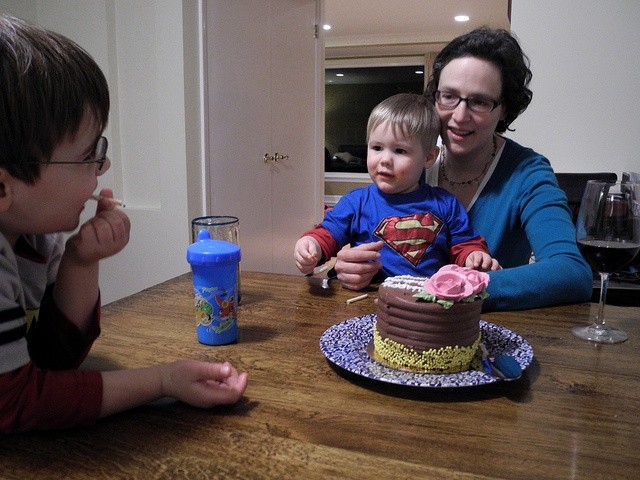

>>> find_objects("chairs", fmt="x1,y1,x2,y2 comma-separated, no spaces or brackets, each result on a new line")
548,172,617,226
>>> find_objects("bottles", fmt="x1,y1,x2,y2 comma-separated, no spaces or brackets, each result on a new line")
186,229,242,346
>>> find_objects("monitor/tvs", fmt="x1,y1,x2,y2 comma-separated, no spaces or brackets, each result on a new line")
323,55,425,205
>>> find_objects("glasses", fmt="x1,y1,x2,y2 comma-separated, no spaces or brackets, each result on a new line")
39,135,109,173
433,90,503,114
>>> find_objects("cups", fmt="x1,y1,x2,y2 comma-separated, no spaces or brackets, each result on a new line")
186,215,244,346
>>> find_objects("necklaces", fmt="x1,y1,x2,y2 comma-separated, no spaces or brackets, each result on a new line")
441,135,498,186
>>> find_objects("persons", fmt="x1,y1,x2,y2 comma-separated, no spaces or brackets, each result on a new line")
294,94,502,274
0,15,249,433
327,26,593,313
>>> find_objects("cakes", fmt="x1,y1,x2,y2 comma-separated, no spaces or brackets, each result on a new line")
370,263,491,374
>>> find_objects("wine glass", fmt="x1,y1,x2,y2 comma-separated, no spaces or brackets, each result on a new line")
568,180,640,345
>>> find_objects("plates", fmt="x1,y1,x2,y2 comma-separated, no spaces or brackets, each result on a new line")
318,302,537,390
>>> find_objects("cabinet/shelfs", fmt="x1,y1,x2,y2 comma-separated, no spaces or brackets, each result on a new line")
198,1,323,275
